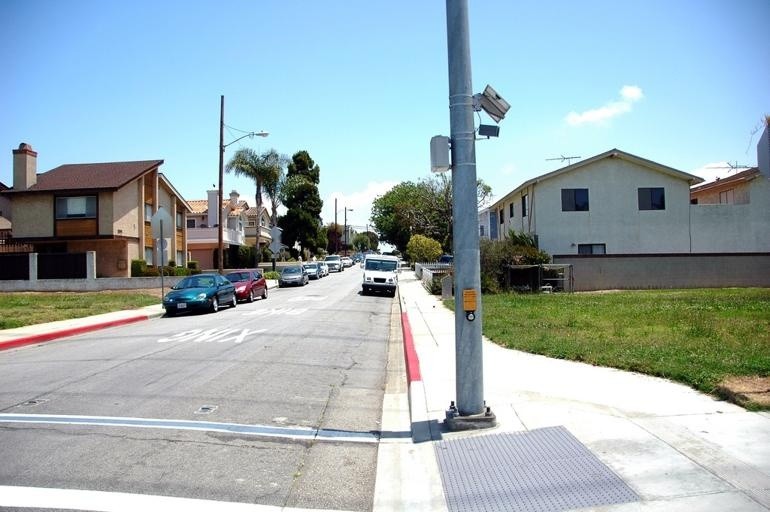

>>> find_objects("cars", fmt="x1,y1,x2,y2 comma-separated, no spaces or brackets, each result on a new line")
162,272,240,317
440,254,453,263
225,269,269,302
278,250,368,288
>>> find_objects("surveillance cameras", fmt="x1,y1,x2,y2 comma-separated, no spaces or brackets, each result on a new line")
479,125,500,136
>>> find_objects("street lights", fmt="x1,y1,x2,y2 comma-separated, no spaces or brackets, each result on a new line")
217,93,272,275
344,207,355,258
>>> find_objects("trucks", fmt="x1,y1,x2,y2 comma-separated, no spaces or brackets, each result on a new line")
359,253,399,297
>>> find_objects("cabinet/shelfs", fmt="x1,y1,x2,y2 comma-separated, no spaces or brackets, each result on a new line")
509,259,576,294
